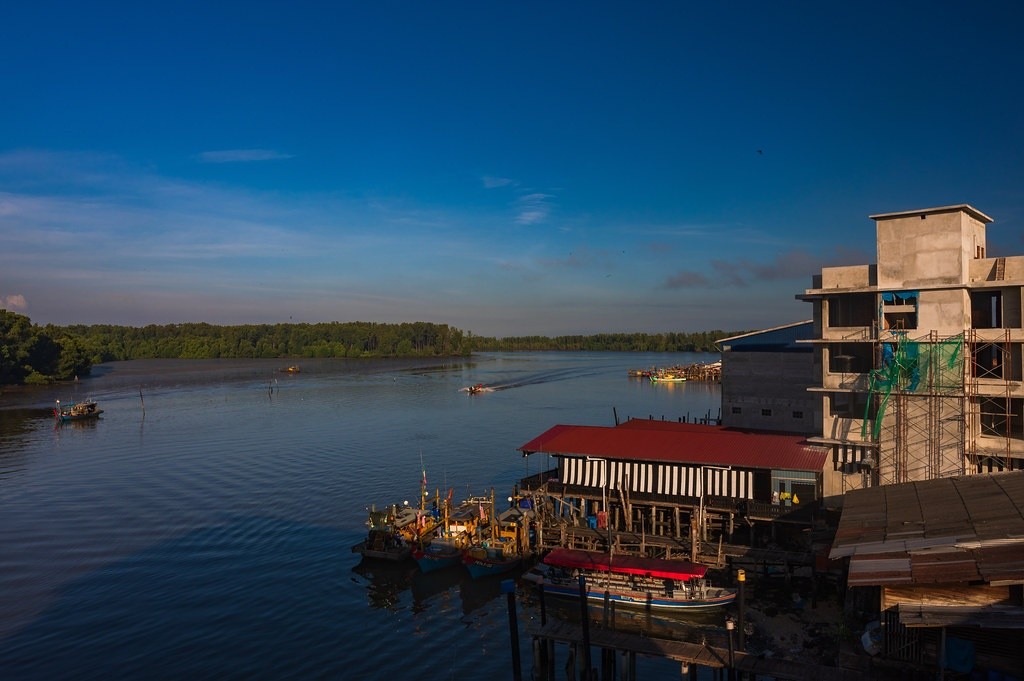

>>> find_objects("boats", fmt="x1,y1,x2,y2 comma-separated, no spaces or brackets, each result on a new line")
532,549,740,612
349,488,549,578
649,373,687,384
54,401,104,420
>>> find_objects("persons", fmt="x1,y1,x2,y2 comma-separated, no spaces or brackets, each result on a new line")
368,529,410,552
432,515,495,553
